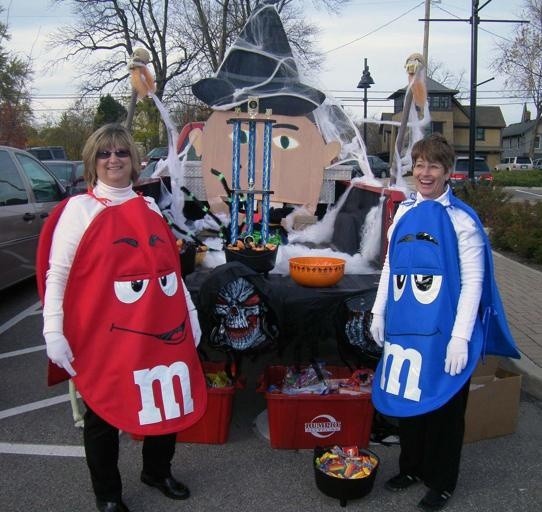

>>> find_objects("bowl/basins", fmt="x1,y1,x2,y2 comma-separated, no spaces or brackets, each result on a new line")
289,256,346,287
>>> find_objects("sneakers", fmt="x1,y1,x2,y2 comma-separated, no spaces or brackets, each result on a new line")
416,488,456,512
384,472,422,492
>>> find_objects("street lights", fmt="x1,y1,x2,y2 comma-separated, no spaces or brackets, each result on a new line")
353,57,374,151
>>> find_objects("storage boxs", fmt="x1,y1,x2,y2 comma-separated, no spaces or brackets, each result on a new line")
127,353,240,446
463,356,522,445
263,364,374,450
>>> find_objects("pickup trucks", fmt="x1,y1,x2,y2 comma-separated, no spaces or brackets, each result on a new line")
158,115,409,368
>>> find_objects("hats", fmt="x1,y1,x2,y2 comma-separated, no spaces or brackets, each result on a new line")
188,3,326,117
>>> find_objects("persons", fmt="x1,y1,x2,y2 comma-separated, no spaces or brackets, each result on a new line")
34,123,211,511
367,131,523,511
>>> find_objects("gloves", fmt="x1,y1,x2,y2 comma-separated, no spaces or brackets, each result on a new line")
367,314,388,349
40,333,81,380
443,337,469,376
185,309,202,352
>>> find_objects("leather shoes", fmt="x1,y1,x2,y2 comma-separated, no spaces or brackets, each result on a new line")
140,469,191,501
95,498,131,512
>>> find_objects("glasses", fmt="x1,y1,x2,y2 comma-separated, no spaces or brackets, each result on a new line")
95,149,131,160
415,162,446,170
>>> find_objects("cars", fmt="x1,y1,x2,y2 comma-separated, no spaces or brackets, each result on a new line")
0,144,71,304
24,144,172,198
341,140,542,193
